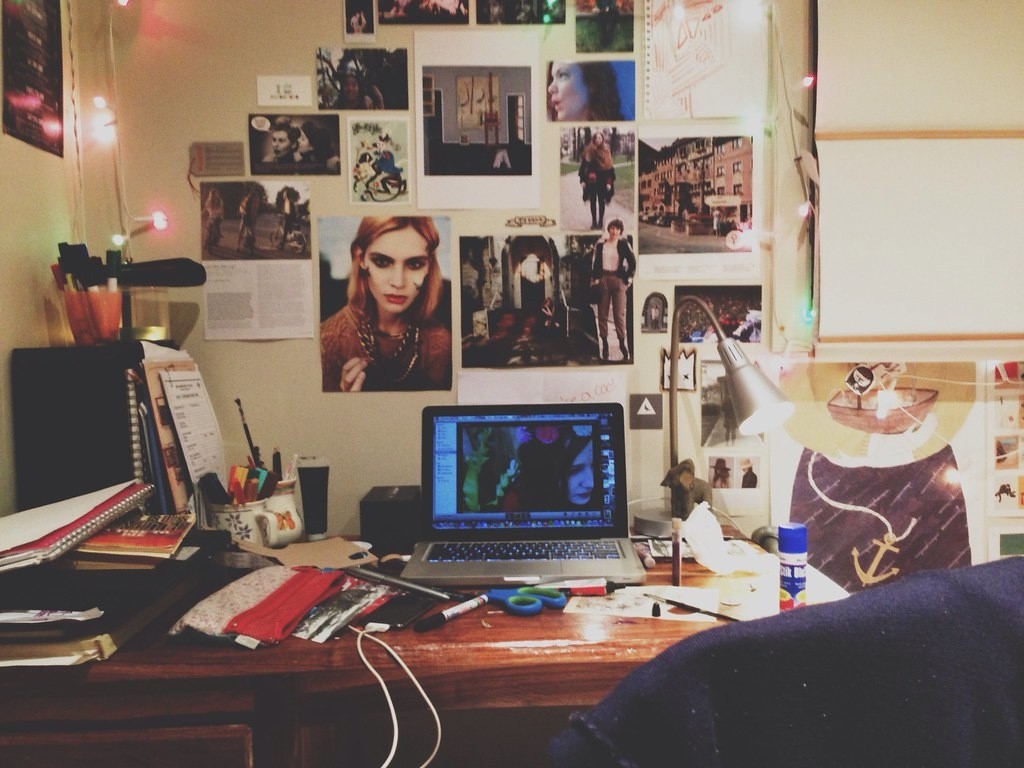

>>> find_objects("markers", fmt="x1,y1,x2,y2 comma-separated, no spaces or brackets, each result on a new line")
45,241,124,295
201,460,269,505
412,593,490,632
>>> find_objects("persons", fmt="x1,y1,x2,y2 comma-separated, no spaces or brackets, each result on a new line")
321,217,452,391
236,190,259,253
710,458,733,488
502,428,594,512
712,207,724,237
592,220,636,360
276,191,300,252
740,458,757,488
333,56,383,110
547,61,621,121
540,298,560,330
578,133,616,230
263,118,331,172
201,188,225,250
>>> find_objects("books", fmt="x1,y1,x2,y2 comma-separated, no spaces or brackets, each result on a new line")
0,479,199,671
124,341,227,517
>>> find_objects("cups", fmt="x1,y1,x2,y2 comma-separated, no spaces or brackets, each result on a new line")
299,466,330,541
259,476,304,545
64,291,120,346
210,499,278,551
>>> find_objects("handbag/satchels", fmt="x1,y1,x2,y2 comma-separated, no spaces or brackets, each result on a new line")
590,283,600,304
166,564,346,646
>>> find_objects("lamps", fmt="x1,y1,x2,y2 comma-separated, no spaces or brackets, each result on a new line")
634,296,796,539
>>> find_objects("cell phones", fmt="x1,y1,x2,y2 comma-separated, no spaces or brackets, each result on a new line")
355,595,438,630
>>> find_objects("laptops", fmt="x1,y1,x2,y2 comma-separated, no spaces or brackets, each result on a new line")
399,403,646,584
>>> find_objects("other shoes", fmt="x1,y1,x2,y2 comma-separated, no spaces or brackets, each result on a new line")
603,337,610,359
619,339,628,357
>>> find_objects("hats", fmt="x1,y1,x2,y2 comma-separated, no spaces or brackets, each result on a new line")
738,458,754,469
710,459,731,471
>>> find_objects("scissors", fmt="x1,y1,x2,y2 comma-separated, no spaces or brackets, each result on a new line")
438,584,567,617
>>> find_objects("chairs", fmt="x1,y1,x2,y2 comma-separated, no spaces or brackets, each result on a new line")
530,555,1024,768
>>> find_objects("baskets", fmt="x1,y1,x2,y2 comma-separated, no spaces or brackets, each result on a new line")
827,385,938,435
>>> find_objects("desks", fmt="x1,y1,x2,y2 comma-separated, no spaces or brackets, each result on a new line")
0,525,849,768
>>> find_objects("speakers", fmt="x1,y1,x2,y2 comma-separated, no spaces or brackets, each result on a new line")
298,466,330,542
358,485,423,563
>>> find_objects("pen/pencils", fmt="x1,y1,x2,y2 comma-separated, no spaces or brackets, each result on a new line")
344,563,451,601
243,446,299,486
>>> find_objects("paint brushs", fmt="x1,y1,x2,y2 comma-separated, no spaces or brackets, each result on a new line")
237,398,263,468
643,593,742,625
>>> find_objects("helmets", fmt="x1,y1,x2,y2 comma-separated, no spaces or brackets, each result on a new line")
335,59,365,78
